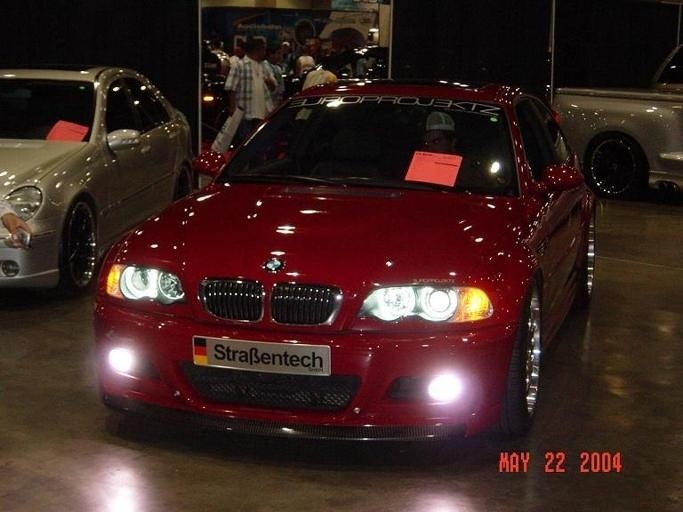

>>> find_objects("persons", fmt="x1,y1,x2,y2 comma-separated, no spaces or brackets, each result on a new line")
201,37,373,153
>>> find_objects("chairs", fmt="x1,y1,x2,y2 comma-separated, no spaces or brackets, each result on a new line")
309,124,381,185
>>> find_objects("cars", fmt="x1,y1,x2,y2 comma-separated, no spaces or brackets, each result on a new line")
96,81,602,443
0,67,199,295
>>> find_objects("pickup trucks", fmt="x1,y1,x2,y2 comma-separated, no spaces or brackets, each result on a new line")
553,45,682,198
